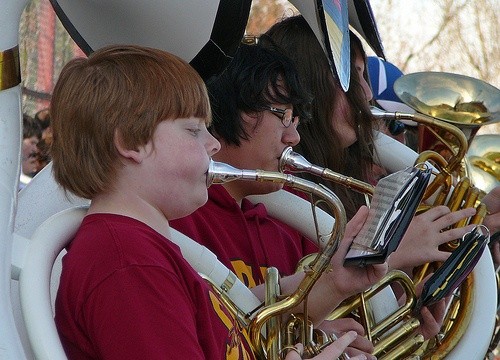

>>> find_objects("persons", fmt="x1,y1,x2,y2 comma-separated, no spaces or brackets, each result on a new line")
18,108,52,193
52,45,388,360
168,13,499,360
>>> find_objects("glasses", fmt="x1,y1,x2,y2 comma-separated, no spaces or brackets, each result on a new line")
253,104,300,128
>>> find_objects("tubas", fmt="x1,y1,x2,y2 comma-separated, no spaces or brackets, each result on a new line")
361,66,500,216
207,157,427,360
278,146,490,360
